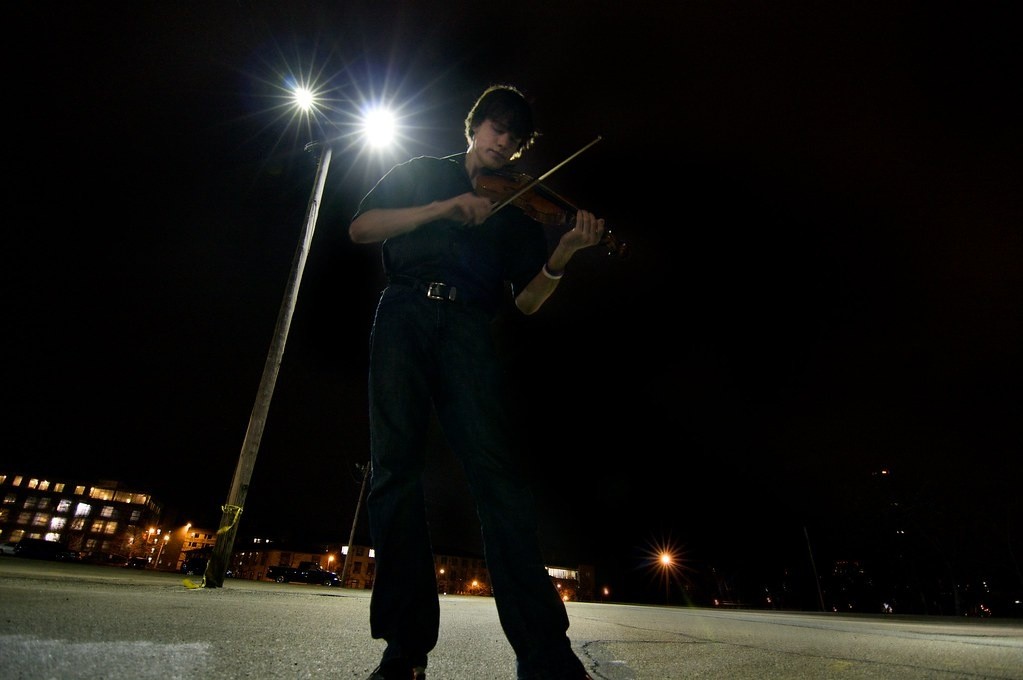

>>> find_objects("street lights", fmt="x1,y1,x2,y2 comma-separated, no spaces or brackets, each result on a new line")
201,85,398,593
660,552,673,605
153,535,171,569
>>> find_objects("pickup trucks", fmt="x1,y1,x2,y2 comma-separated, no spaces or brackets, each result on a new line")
266,562,342,587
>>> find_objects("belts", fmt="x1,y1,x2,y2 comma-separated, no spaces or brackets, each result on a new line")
385,275,472,304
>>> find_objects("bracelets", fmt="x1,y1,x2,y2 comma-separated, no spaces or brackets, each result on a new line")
542,261,566,280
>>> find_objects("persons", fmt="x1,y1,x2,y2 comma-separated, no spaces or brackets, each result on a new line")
349,86,606,680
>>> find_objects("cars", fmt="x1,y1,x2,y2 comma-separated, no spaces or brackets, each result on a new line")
0,537,148,570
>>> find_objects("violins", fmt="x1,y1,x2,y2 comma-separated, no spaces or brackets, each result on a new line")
474,168,627,258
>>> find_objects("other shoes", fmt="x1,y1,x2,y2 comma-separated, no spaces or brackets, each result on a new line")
518,654,592,680
364,655,429,680
